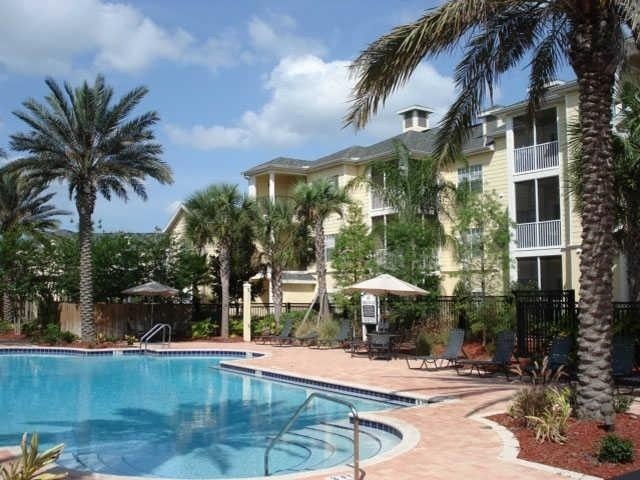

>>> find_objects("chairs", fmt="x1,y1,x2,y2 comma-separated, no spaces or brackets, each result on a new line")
347,331,409,360
251,318,353,350
404,327,635,395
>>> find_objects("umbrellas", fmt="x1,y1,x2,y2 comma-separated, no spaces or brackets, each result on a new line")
122,280,180,329
344,272,432,334
